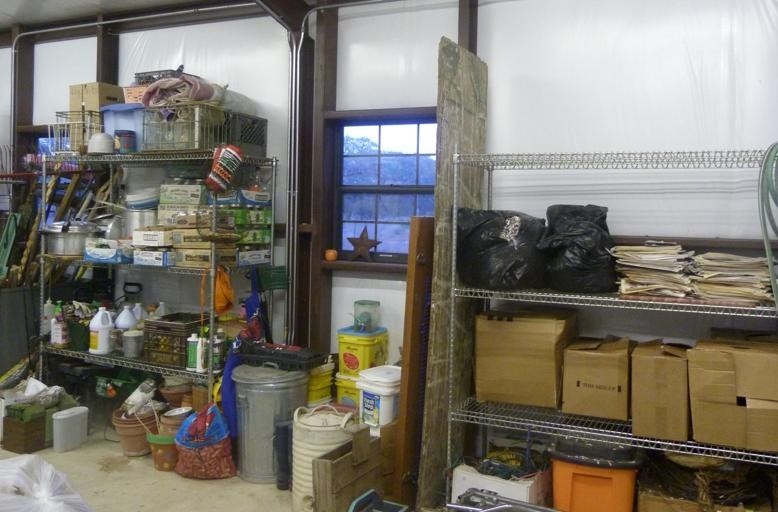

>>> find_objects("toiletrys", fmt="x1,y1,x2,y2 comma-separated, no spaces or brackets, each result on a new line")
49,299,69,345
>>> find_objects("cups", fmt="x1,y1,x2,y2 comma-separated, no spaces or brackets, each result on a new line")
123,330,144,361
353,299,379,333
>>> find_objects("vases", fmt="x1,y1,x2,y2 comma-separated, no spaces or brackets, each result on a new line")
112,375,193,472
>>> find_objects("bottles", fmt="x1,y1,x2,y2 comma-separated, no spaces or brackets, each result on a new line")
185,326,227,375
165,172,205,185
253,166,273,194
195,185,253,243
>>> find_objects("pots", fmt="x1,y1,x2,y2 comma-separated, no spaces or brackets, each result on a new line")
92,197,158,239
43,216,98,257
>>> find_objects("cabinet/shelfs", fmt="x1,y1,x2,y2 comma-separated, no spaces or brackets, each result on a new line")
0,278,106,378
448,149,777,512
36,149,278,450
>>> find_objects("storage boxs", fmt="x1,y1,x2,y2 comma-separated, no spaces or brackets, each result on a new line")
450,441,778,512
561,335,637,419
629,338,694,441
474,309,582,409
101,100,146,150
69,80,126,150
684,323,778,453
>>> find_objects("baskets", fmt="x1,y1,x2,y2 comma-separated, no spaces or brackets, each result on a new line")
140,102,232,152
214,111,267,157
135,71,200,85
122,84,150,104
142,312,219,368
47,111,104,155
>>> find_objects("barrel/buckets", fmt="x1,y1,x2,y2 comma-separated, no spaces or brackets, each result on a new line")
154,301,171,316
290,406,352,510
230,363,307,485
86,306,115,355
124,209,156,238
113,304,137,351
132,300,150,322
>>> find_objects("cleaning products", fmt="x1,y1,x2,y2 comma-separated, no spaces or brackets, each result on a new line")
186,328,226,374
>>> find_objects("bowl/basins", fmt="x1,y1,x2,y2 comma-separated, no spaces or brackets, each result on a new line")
163,406,193,420
87,131,115,154
